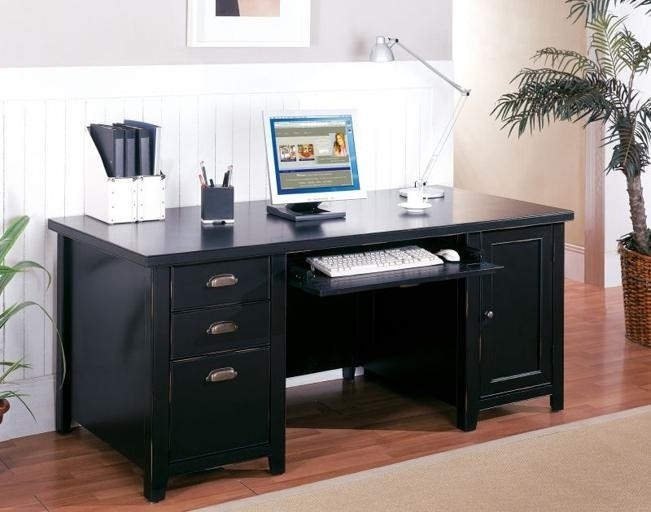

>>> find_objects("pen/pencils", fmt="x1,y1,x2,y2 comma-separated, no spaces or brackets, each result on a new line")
198,161,233,187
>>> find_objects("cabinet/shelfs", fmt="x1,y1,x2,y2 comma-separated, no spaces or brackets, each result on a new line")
359,223,565,431
53,235,292,501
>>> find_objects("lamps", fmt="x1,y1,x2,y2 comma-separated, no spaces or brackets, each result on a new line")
369,34,472,199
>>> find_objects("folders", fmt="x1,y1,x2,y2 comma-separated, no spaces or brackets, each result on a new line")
91,119,162,177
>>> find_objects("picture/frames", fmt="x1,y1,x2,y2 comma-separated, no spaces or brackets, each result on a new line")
185,1,311,49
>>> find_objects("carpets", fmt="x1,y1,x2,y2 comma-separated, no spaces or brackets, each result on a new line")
186,405,651,511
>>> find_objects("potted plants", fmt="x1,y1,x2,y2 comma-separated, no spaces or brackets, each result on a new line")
1,215,66,432
490,0,651,348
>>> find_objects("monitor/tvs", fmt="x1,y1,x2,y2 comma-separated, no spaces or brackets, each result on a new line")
261,106,370,223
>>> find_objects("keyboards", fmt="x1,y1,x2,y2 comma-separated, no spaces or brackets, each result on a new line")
305,244,444,278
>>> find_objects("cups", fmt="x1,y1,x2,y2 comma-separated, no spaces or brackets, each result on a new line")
407,190,428,208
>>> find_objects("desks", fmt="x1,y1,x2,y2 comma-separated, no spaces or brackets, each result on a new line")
45,185,574,503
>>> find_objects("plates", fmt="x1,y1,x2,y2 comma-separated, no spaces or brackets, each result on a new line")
398,202,431,213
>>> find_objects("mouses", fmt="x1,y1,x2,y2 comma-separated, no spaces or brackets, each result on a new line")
435,248,461,262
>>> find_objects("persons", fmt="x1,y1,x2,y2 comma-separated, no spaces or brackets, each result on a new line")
334,133,346,156
